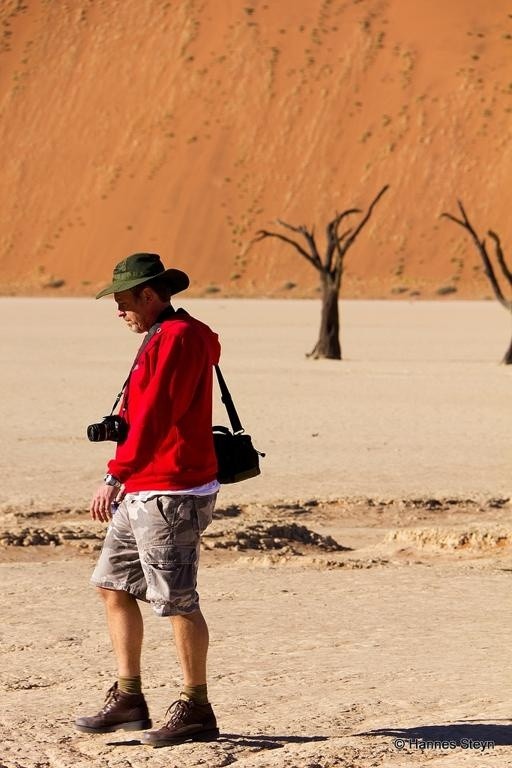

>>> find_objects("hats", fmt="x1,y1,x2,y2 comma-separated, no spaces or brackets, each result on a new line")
96,253,189,300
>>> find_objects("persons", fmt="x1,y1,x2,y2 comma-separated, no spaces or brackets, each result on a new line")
72,252,222,747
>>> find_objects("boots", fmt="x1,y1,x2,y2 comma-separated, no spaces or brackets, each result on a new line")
76,689,152,733
141,699,218,744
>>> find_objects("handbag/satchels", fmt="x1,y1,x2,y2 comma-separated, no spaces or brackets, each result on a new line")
212,426,261,484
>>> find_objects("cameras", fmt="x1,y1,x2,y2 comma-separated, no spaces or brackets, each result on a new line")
87,415,126,442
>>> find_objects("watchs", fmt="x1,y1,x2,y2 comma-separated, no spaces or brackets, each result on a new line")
104,474,120,488
